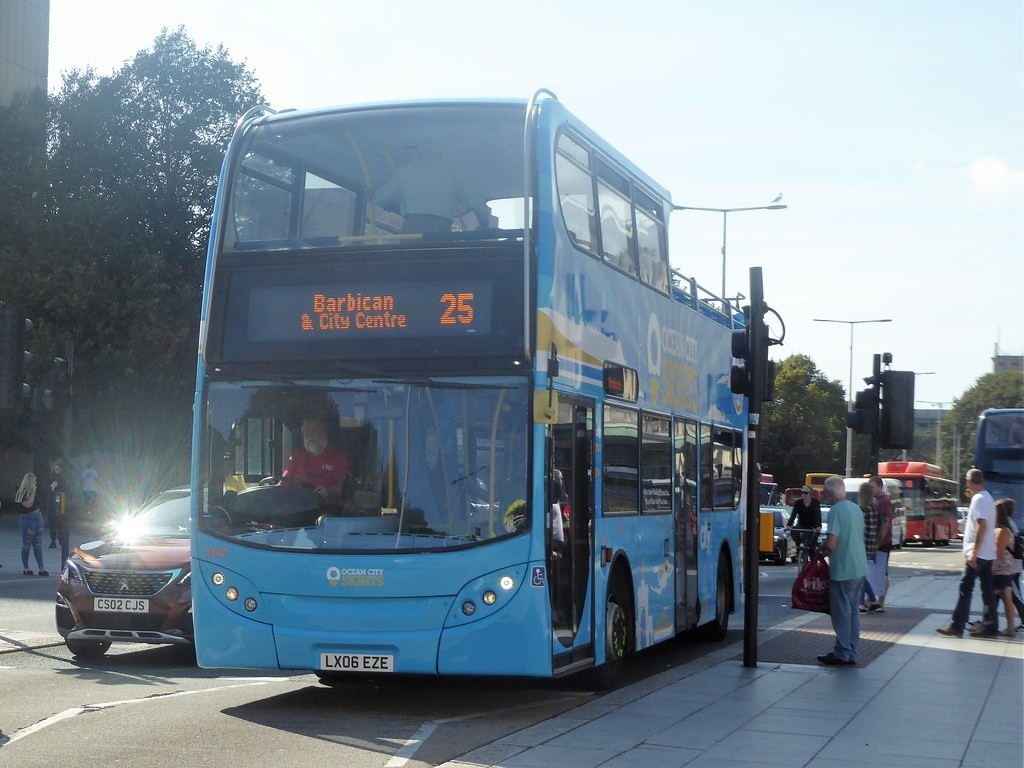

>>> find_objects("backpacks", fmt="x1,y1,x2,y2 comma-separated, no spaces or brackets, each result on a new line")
1000,526,1024,559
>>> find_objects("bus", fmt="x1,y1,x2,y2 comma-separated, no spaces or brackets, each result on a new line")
876,461,959,546
975,408,1024,532
805,472,838,492
188,86,745,694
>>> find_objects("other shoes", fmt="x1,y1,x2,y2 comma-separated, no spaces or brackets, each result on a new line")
817,652,855,664
935,625,962,637
57,535,63,545
49,542,56,548
39,570,49,576
23,569,33,575
999,629,1015,636
858,605,866,612
970,627,997,639
875,607,886,612
868,604,880,611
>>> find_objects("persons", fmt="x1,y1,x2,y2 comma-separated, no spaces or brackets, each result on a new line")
785,476,892,665
15,461,97,576
935,469,1024,641
277,418,349,506
547,468,571,556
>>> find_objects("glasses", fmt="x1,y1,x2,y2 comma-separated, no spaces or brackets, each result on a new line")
800,491,810,494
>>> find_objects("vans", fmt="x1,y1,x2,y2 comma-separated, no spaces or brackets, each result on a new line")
841,477,908,546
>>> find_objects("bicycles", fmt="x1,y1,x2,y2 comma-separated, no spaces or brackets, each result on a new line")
782,527,818,575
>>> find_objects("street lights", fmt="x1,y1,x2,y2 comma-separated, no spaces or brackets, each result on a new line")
672,204,788,312
813,318,895,478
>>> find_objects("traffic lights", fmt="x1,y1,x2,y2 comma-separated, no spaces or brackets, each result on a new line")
731,326,752,398
845,387,877,435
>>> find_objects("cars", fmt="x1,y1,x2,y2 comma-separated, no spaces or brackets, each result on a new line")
956,506,969,534
55,482,271,657
759,507,831,565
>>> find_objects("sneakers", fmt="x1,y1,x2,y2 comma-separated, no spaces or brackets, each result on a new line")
1014,624,1024,634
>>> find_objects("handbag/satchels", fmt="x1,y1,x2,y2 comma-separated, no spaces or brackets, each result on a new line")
790,551,830,612
